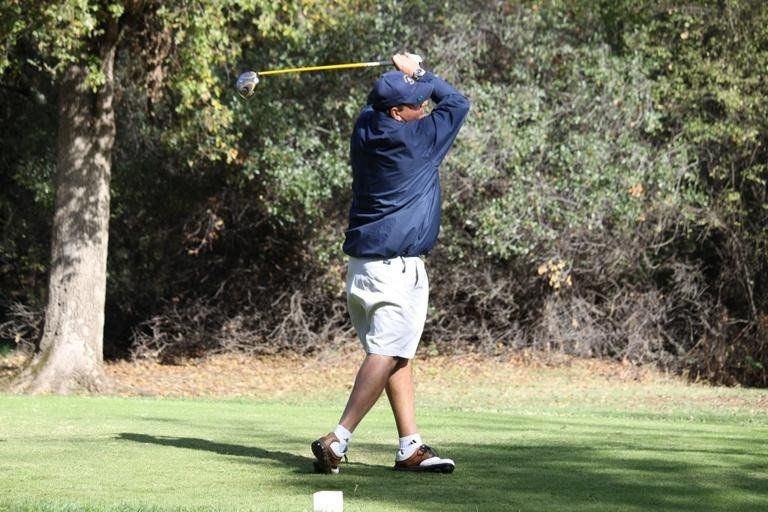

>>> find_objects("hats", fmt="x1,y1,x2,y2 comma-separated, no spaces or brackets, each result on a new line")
372,70,434,111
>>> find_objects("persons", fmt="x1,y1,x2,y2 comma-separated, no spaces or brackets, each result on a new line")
309,52,471,475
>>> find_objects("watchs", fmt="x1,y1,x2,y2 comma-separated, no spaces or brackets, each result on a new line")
412,68,425,79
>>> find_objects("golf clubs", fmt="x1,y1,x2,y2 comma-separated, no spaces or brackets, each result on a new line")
235,55,423,101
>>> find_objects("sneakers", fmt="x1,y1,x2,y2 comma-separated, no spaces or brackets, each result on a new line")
391,444,455,473
311,431,349,473
